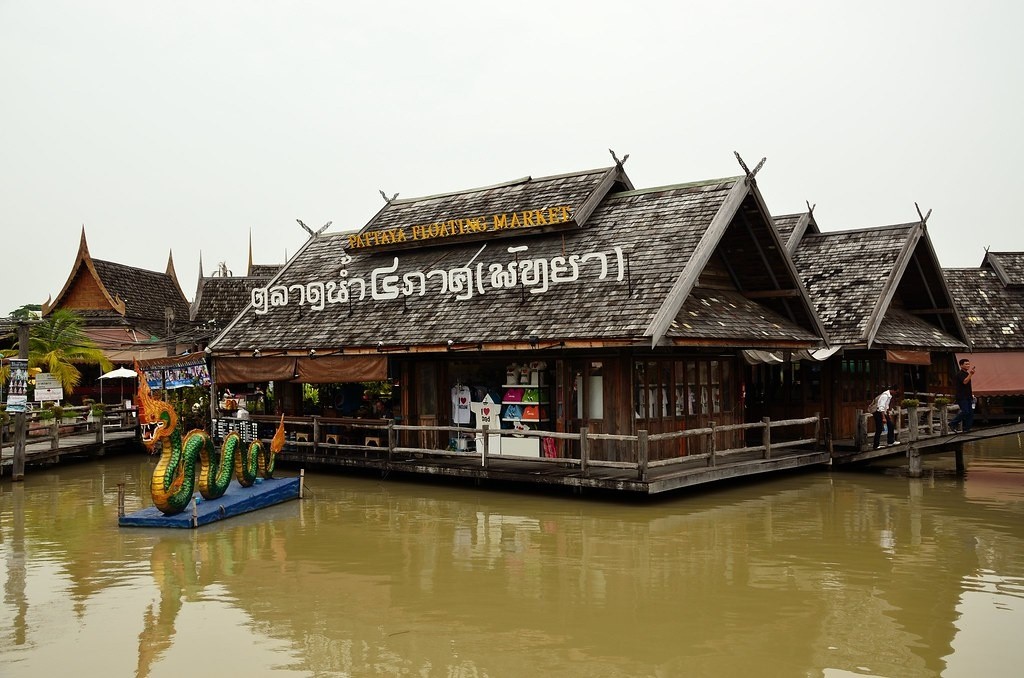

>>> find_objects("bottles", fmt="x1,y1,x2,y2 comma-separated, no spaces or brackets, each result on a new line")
971,398,976,409
884,423,888,432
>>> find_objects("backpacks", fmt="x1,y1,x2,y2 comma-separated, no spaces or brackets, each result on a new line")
867,393,889,413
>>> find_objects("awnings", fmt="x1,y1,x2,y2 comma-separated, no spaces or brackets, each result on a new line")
955,352,1024,397
56,344,195,366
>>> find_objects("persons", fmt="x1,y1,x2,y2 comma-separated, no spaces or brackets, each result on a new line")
949,358,977,434
872,384,900,450
372,399,392,420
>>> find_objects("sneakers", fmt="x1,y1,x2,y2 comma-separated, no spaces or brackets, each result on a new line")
888,441,900,446
875,446,880,449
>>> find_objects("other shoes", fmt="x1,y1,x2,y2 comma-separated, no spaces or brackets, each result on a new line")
949,423,957,434
962,428,969,431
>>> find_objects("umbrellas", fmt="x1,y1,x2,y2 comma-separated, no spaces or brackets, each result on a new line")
97,365,138,379
